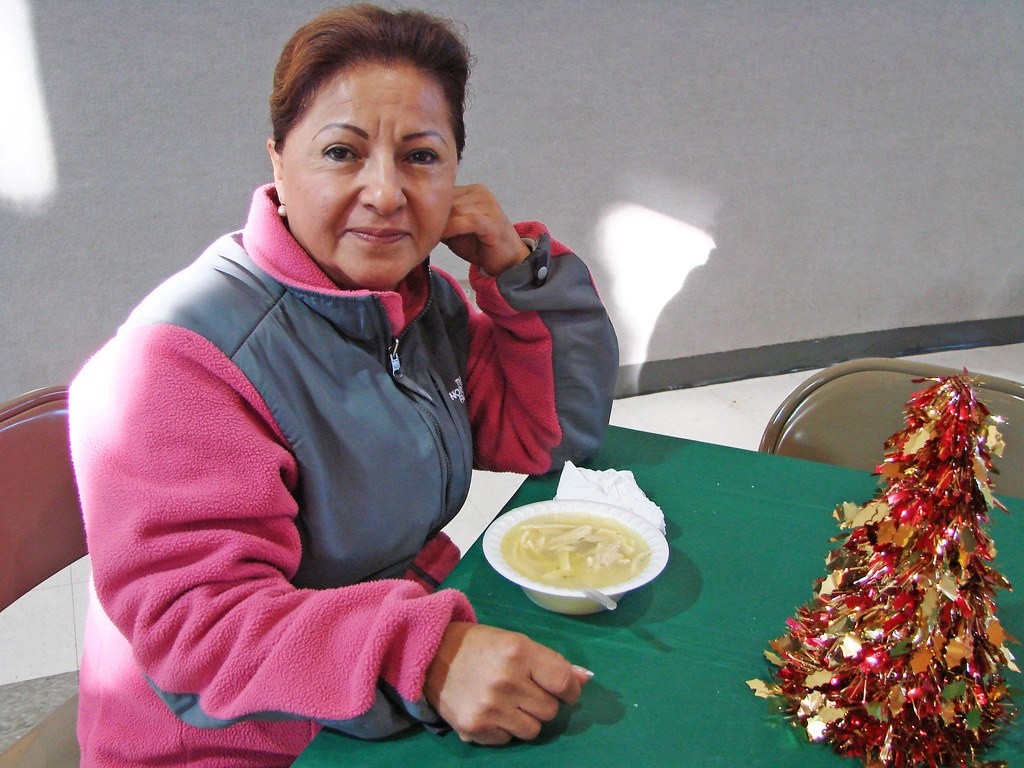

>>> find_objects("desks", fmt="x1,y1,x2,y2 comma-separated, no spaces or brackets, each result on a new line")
284,424,1024,768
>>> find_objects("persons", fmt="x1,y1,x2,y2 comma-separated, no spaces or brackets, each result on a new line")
64,2,621,768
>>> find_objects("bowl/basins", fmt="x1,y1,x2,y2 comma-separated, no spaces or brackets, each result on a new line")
483,499,669,615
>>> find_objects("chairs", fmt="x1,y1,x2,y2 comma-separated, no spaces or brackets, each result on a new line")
759,357,1024,500
0,382,85,768
